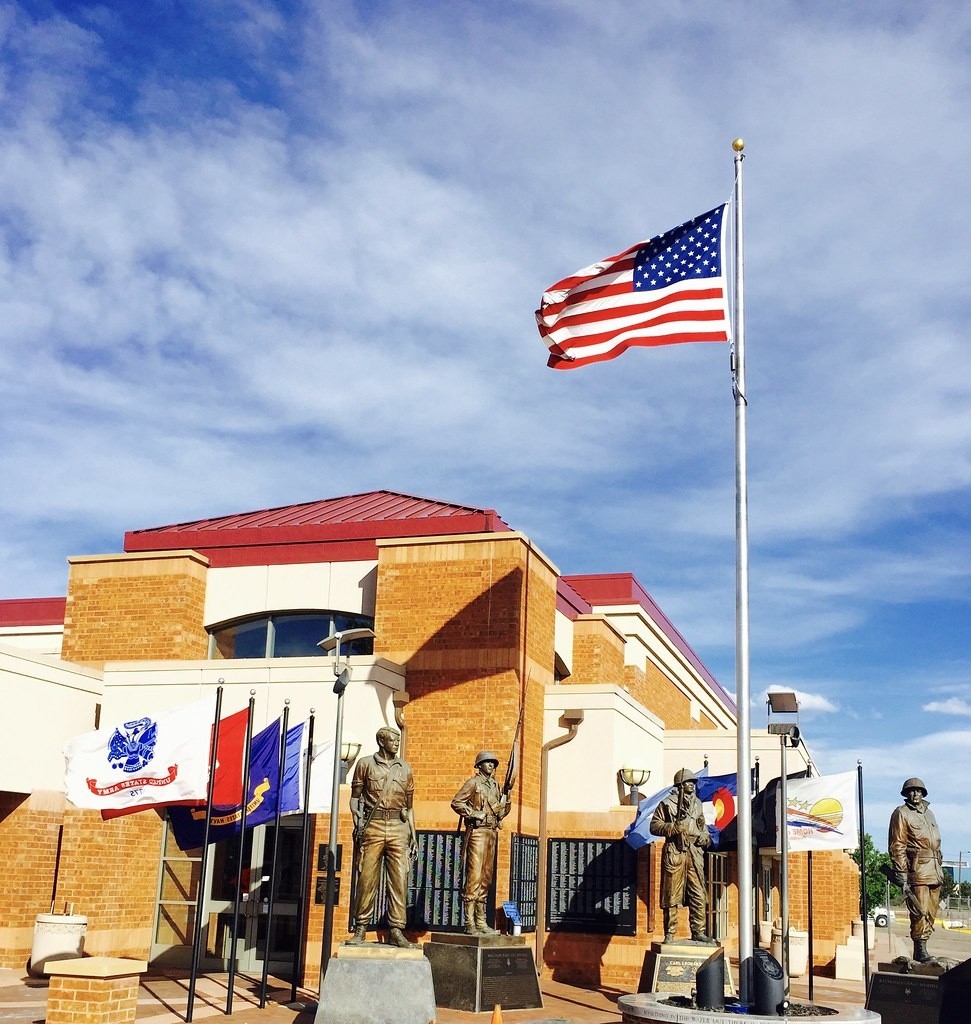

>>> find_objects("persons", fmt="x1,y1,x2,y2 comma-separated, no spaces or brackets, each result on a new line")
887,778,945,962
450,751,512,935
650,769,716,942
350,726,418,948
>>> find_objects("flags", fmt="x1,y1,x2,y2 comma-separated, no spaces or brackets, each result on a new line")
284,722,310,814
776,769,861,852
65,693,216,810
716,771,809,850
166,714,282,850
694,769,756,848
535,202,730,369
100,707,250,821
624,767,708,850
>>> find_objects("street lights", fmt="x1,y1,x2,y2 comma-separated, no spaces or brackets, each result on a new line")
315,628,379,998
764,689,802,1012
959,850,971,910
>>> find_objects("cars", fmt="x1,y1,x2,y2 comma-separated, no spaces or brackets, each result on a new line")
872,899,895,929
